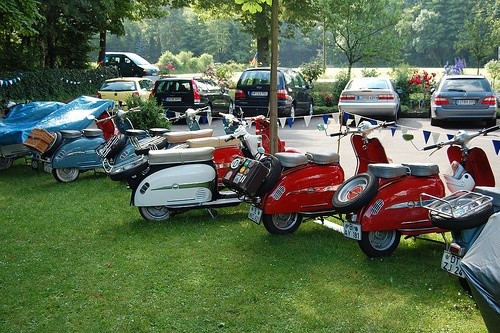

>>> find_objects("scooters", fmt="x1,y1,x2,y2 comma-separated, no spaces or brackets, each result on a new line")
417,186,499,333
330,126,500,254
221,120,397,236
131,111,264,222
0,101,65,170
99,105,210,187
23,105,141,184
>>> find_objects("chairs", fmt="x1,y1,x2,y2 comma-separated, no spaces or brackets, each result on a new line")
178,85,188,92
168,85,176,92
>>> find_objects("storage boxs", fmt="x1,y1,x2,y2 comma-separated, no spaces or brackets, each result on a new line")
23,129,54,153
222,155,269,195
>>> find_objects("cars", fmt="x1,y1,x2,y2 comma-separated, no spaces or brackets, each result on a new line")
104,50,159,77
338,76,400,126
97,76,153,110
429,74,497,126
234,67,315,120
147,81,234,121
